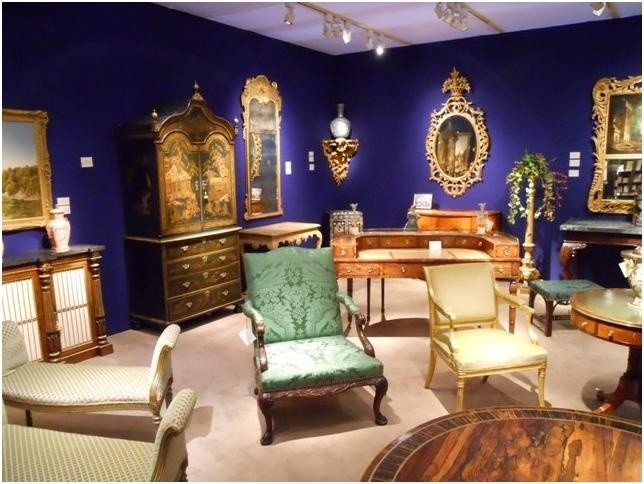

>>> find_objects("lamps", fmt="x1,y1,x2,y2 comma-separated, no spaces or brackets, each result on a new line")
589,1,620,19
433,2,506,36
283,2,414,56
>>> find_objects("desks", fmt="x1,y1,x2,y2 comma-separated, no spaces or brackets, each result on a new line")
239,220,324,250
360,404,642,482
331,229,520,337
558,216,641,282
570,287,641,416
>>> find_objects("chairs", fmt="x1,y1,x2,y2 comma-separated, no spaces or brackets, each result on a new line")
423,261,547,414
240,247,391,445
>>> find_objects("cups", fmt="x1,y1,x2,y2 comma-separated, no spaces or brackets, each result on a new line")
428,240,442,250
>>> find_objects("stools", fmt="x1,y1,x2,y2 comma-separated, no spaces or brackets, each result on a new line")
527,278,607,337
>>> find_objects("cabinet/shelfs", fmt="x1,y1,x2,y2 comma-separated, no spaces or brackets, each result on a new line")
1,244,115,363
114,80,243,330
322,209,363,244
412,208,502,231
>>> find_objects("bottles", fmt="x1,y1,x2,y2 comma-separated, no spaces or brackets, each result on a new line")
474,202,490,234
347,202,362,235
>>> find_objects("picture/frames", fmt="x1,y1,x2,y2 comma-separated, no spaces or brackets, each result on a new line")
586,74,642,217
239,73,283,220
424,67,490,198
1,106,54,234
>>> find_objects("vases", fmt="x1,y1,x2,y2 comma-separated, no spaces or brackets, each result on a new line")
329,101,351,138
46,207,71,253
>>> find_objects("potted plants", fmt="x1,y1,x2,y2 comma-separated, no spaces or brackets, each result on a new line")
504,151,567,294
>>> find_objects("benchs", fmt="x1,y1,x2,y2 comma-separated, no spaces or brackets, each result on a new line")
2,319,180,435
2,389,195,481
